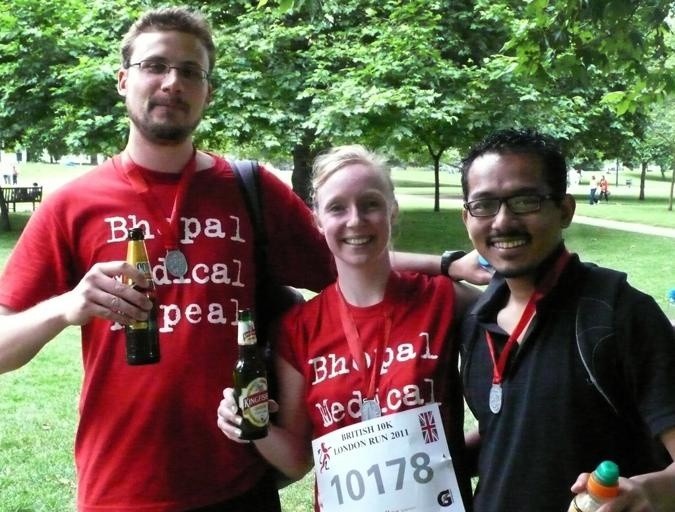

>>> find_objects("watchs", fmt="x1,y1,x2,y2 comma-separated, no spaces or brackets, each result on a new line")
441,248,466,281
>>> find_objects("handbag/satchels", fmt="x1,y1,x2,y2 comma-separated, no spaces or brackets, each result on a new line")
228,155,305,421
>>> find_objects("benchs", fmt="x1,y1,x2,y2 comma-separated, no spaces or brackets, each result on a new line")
2,186,42,212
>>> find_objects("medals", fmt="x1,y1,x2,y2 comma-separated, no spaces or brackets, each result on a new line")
360,397,381,422
165,247,189,277
488,382,503,416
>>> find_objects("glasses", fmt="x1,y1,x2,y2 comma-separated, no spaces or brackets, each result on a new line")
464,192,560,218
126,59,209,82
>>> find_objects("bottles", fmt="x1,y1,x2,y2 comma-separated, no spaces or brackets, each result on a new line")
122,229,160,367
235,306,269,441
566,462,620,512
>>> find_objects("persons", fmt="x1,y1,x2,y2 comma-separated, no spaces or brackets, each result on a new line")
1,7,497,511
589,175,601,208
457,126,674,512
11,164,18,185
597,174,610,204
214,140,485,511
